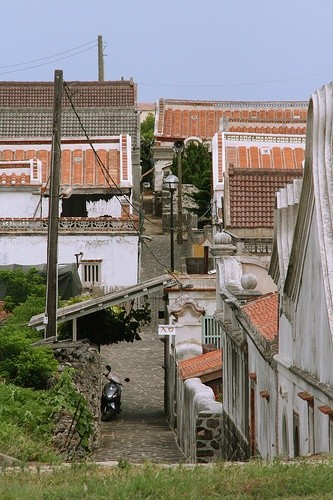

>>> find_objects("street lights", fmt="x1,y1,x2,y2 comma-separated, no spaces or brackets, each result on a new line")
165,174,180,272
46,186,73,337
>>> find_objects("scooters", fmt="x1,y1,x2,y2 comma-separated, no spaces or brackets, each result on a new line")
100,364,130,421
